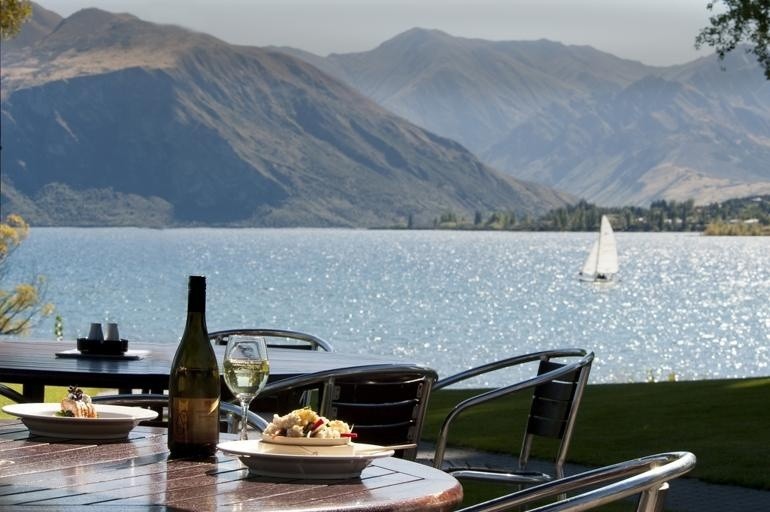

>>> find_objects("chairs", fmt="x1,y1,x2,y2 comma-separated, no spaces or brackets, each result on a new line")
209,329,335,352
90,347,695,512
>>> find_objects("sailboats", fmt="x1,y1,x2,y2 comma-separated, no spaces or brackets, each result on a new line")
578,213,619,285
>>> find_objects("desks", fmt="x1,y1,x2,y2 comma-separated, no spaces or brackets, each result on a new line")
2,339,427,425
1,424,464,512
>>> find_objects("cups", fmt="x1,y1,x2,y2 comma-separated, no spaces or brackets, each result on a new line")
86,322,103,340
105,323,121,341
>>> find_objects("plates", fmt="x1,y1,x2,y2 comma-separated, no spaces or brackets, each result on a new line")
261,432,352,445
216,440,396,479
3,403,160,440
55,347,150,360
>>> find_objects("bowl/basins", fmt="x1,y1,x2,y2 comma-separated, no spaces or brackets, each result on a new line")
77,338,128,356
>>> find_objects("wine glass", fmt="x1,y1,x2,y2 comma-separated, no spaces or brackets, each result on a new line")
224,336,270,441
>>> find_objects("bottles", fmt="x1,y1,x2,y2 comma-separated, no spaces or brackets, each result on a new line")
165,275,221,455
